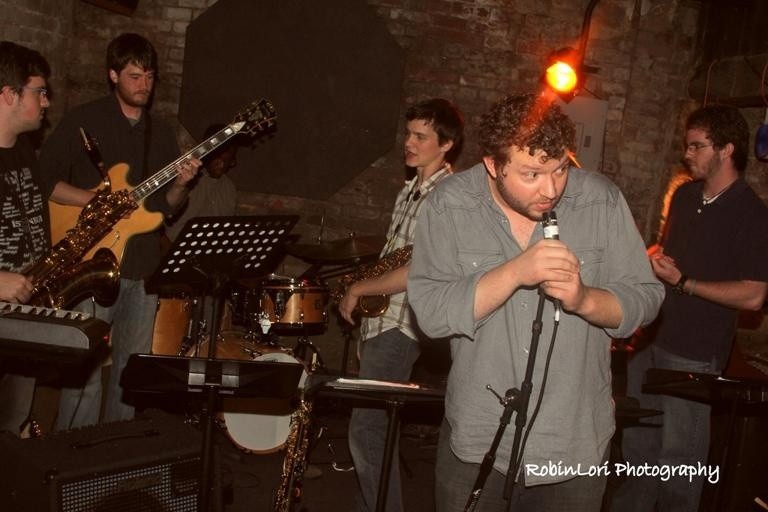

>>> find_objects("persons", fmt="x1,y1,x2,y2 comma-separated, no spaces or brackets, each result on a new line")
612,98,768,510
0,39,103,437
42,34,202,432
160,124,236,258
325,96,465,512
405,96,666,510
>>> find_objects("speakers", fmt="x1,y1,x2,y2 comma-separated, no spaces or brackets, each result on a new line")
2,417,205,512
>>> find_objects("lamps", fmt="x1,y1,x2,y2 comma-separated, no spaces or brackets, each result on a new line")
544,1,599,94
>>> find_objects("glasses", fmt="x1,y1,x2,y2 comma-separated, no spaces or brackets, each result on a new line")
21,84,48,95
684,140,726,150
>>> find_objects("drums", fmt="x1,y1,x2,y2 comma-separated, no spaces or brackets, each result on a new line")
231,277,265,326
144,274,194,356
253,276,329,336
178,329,314,454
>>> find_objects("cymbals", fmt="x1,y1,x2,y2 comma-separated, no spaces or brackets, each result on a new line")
286,230,385,261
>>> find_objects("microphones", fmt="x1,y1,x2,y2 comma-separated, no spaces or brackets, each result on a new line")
542,211,564,321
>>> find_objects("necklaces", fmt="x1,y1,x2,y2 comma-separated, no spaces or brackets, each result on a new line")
700,186,727,206
386,166,445,249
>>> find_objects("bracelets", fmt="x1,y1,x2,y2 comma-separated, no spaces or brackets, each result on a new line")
688,279,697,296
670,274,688,295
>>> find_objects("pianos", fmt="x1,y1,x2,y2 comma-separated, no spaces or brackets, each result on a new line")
0,300,112,389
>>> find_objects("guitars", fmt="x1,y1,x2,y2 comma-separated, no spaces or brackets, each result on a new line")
48,98,279,266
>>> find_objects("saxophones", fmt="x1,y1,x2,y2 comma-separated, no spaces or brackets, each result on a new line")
19,126,138,308
329,244,414,317
